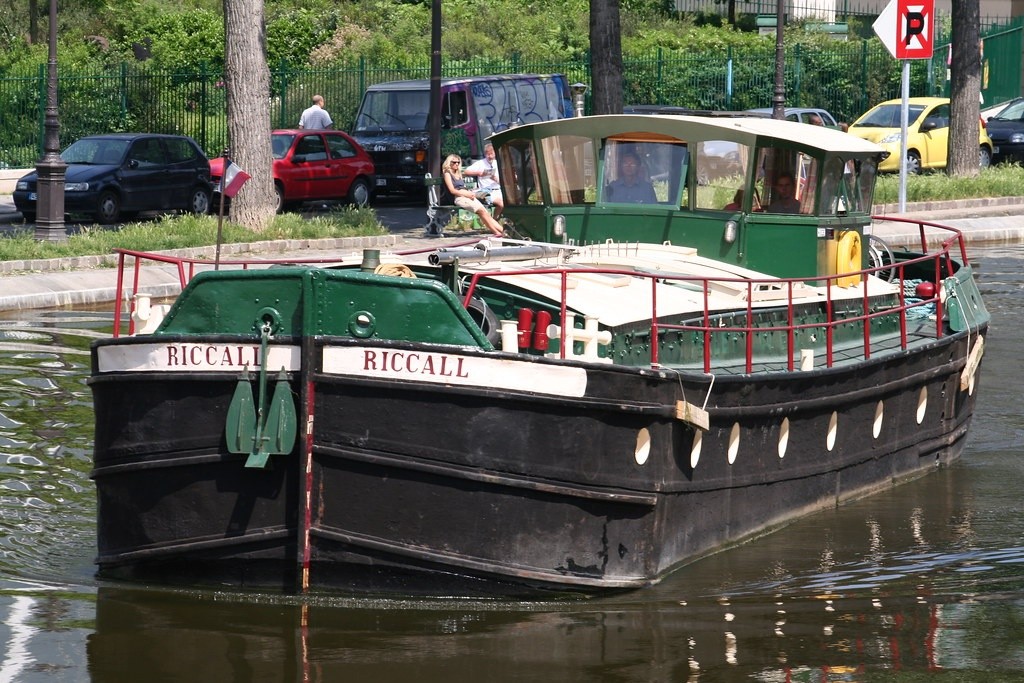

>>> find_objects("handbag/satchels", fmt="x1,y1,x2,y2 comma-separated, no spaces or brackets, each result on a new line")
474,186,493,205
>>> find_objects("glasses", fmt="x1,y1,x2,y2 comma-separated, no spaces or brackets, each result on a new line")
451,161,460,164
490,166,493,171
623,164,638,167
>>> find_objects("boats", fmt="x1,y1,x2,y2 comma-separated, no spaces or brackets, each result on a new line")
85,109,994,594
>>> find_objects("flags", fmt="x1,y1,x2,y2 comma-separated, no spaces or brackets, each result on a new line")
212,160,251,199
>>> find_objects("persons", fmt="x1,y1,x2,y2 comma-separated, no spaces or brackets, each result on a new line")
720,184,748,211
442,153,505,237
603,151,658,206
802,113,856,203
297,94,333,153
765,173,801,215
464,143,505,221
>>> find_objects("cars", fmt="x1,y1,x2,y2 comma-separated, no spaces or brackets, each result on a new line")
706,108,838,177
12,133,214,227
209,128,377,214
621,104,686,116
847,96,993,175
985,96,1024,168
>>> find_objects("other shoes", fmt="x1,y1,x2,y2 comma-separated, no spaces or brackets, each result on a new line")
481,222,486,230
494,233,502,237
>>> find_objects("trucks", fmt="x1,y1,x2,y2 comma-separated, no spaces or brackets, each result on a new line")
347,74,577,203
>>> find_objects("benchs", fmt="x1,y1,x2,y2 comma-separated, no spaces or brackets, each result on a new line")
425,173,503,238
